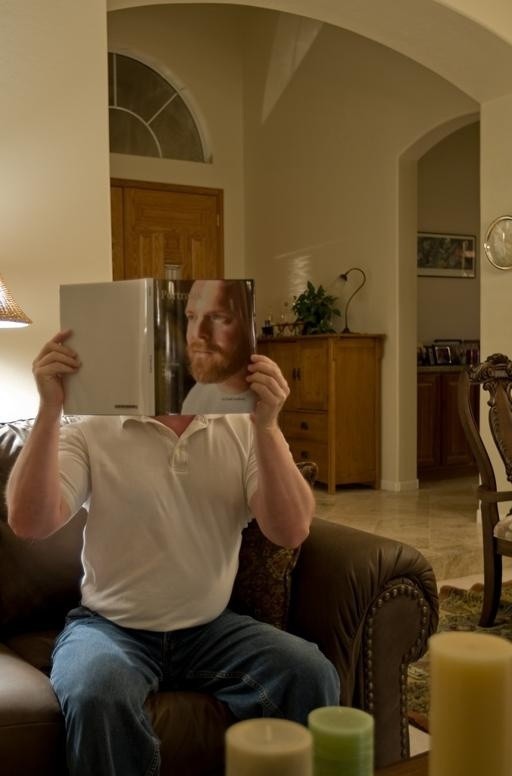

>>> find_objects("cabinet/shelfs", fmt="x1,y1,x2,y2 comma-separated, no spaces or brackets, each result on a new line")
417,372,478,478
257,333,387,493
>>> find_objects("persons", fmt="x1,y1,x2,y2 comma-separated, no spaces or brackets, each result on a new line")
181,279,255,415
4,329,341,776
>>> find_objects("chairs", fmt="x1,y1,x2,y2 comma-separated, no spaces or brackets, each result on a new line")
1,419,441,776
456,354,512,628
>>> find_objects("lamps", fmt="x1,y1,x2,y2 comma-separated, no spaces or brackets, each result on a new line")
1,279,34,331
329,266,366,334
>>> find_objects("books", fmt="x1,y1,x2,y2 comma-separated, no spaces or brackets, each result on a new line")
60,274,261,417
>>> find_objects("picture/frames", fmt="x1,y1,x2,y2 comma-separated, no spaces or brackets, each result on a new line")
416,231,477,278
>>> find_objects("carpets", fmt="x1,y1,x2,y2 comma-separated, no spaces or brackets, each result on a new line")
403,579,512,735
